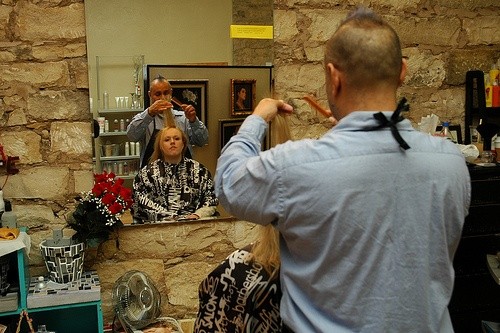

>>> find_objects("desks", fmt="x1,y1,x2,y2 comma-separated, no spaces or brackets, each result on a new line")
1,226,104,333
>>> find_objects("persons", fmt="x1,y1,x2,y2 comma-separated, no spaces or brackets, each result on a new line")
126,75,221,222
234,87,247,110
191,13,469,333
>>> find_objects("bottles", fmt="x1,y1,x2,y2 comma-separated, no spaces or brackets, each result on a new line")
481,152,494,163
105,120,109,133
483,62,500,108
439,122,454,140
125,141,140,156
114,118,130,132
36,276,47,291
103,89,109,110
103,161,129,175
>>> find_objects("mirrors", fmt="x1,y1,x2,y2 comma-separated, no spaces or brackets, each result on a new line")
85,0,273,227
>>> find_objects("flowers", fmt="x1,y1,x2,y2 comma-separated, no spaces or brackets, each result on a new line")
65,170,134,245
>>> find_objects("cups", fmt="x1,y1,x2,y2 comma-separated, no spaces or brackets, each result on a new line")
494,136,500,163
103,143,119,157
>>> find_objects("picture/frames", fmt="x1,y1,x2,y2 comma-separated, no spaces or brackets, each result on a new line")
435,124,461,144
166,78,209,129
219,118,267,159
229,77,255,114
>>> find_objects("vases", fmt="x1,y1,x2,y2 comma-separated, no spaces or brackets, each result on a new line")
39,237,84,283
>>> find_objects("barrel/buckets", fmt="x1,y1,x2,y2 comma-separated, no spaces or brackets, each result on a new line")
39,238,85,283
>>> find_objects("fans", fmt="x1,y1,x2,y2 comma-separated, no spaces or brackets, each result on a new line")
108,269,165,333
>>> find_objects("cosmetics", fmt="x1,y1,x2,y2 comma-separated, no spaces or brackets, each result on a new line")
97,90,140,175
0,190,17,228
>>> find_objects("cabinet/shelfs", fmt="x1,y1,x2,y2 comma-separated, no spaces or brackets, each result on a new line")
445,166,500,333
98,109,143,180
